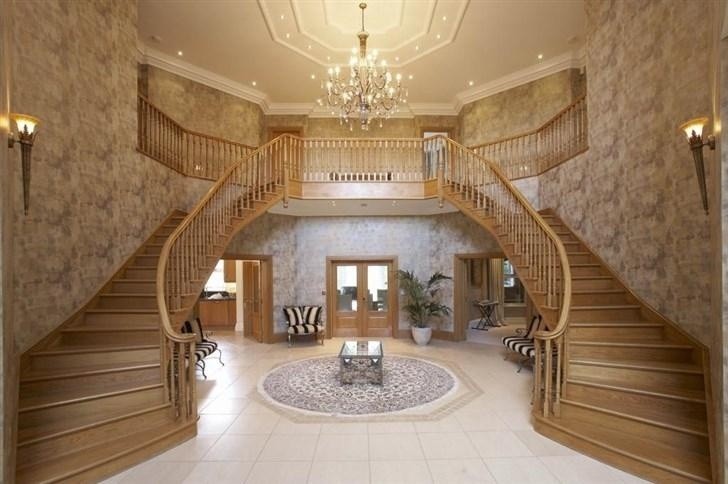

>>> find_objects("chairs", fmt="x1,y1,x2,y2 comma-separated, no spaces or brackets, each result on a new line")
283,304,326,348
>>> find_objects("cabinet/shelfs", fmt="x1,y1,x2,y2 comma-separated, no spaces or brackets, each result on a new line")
200,300,236,330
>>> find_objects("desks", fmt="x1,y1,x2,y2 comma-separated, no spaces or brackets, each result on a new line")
472,300,501,330
337,339,384,385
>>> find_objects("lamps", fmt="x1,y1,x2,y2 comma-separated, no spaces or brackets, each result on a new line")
680,115,715,215
317,1,409,131
7,112,41,215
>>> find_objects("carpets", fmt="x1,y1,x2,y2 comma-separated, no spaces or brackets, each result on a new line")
257,353,459,417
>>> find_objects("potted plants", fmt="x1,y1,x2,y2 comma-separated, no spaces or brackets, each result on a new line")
392,269,455,345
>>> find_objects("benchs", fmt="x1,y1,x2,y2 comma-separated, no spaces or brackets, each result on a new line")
167,316,224,379
501,314,557,373
